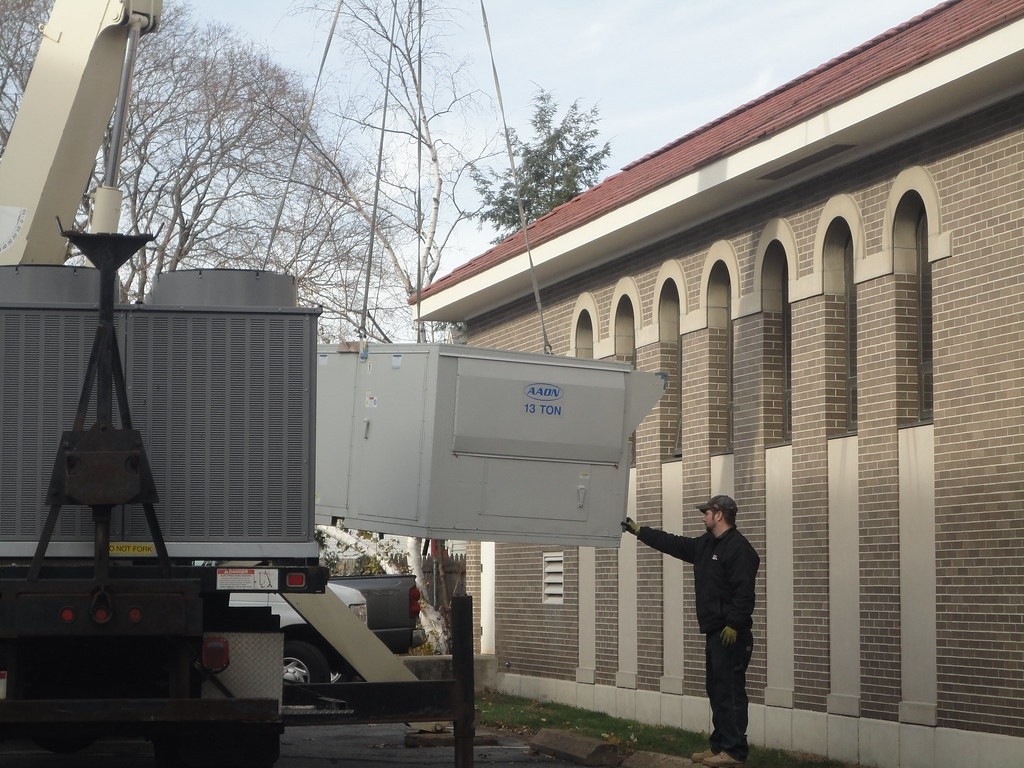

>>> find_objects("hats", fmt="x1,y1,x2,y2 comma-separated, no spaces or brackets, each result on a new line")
695,495,738,515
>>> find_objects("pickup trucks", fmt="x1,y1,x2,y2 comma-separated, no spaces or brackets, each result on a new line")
226,572,428,685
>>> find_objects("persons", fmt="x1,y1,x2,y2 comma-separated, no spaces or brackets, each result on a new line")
621,494,761,767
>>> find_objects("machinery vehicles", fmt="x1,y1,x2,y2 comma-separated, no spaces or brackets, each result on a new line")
0,2,333,768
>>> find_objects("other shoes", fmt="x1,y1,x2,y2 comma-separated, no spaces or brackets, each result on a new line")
690,748,714,763
702,751,744,768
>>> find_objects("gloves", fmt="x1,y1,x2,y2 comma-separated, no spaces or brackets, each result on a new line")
621,517,640,536
720,626,736,646
